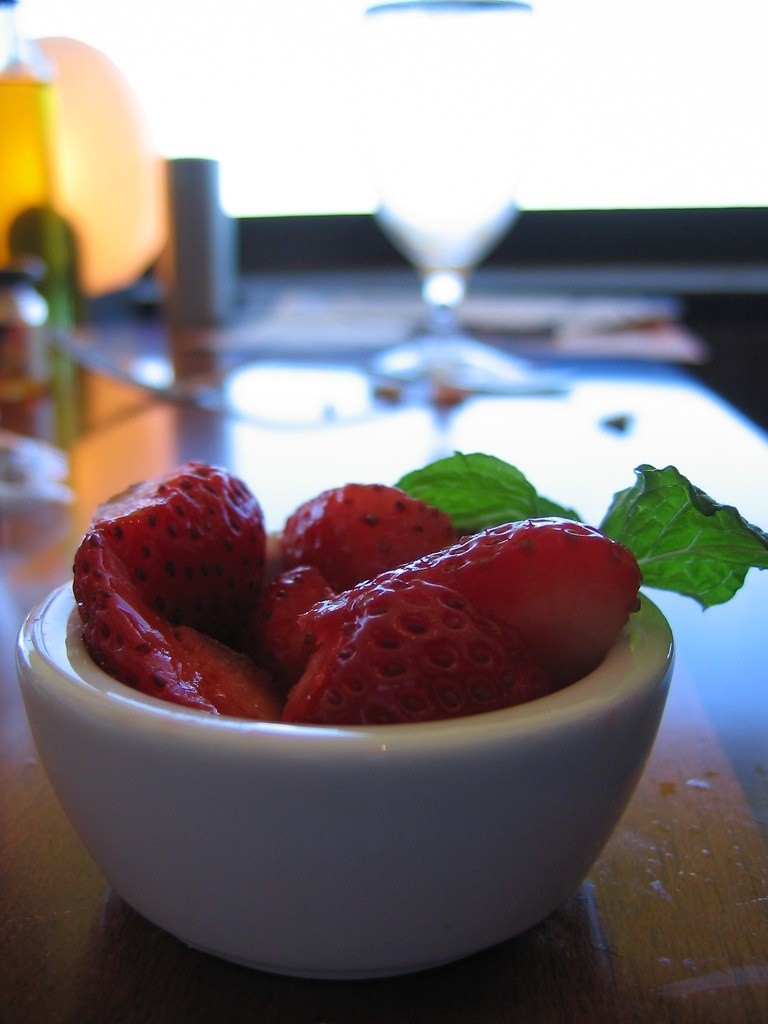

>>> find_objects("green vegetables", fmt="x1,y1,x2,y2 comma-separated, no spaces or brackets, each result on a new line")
389,452,768,610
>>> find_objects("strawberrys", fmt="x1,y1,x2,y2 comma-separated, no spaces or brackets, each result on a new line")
68,462,642,725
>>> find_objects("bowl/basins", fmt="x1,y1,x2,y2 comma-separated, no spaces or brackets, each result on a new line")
11,536,680,979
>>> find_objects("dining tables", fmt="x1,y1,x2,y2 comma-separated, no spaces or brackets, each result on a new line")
0,322,768,1024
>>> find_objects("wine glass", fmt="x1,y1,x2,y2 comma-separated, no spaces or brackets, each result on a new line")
344,1,576,395
